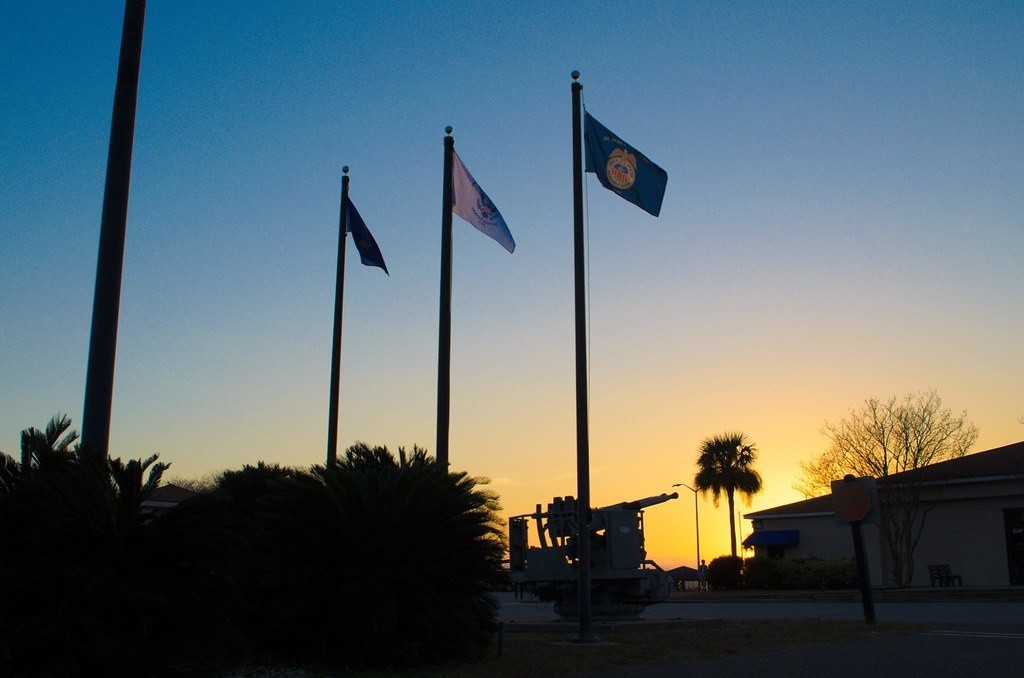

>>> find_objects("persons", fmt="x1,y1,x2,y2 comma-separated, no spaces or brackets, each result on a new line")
698,559,708,592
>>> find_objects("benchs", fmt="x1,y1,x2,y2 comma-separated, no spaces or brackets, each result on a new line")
927,564,963,587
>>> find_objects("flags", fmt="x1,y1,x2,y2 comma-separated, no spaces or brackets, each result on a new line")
451,147,516,254
583,110,668,218
347,196,389,277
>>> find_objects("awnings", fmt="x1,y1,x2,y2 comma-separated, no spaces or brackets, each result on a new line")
742,530,799,545
